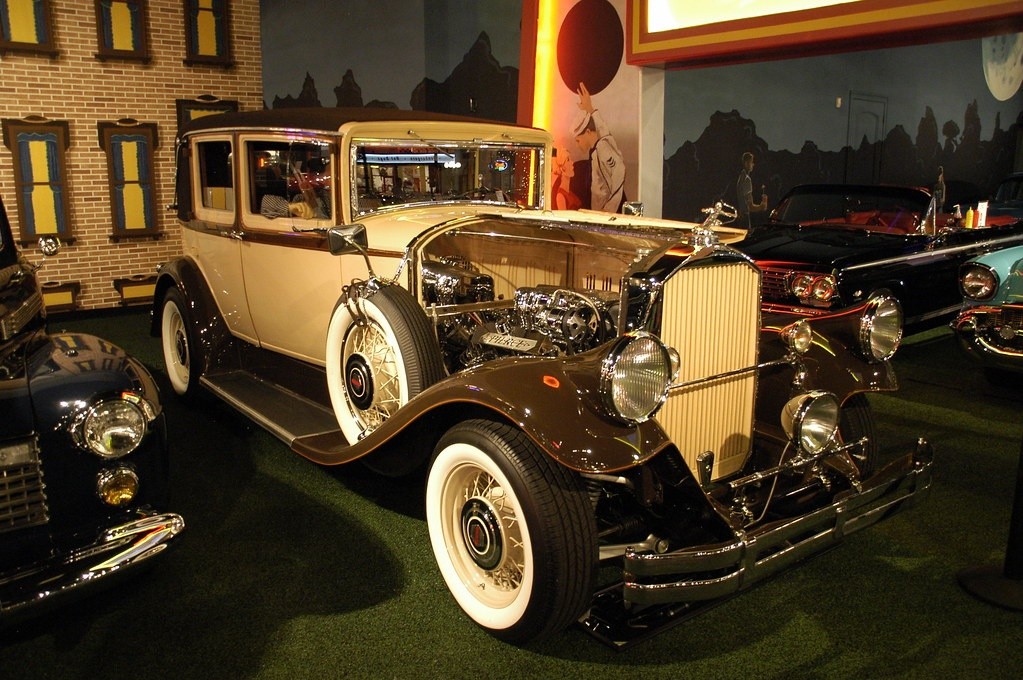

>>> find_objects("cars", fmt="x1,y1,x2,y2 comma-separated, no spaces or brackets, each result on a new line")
1,196,187,635
743,182,1023,401
155,106,938,644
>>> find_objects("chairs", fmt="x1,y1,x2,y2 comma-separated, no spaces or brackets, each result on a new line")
877,210,913,230
358,193,382,217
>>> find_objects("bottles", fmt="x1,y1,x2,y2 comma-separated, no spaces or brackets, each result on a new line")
965,206,980,229
953,204,962,228
